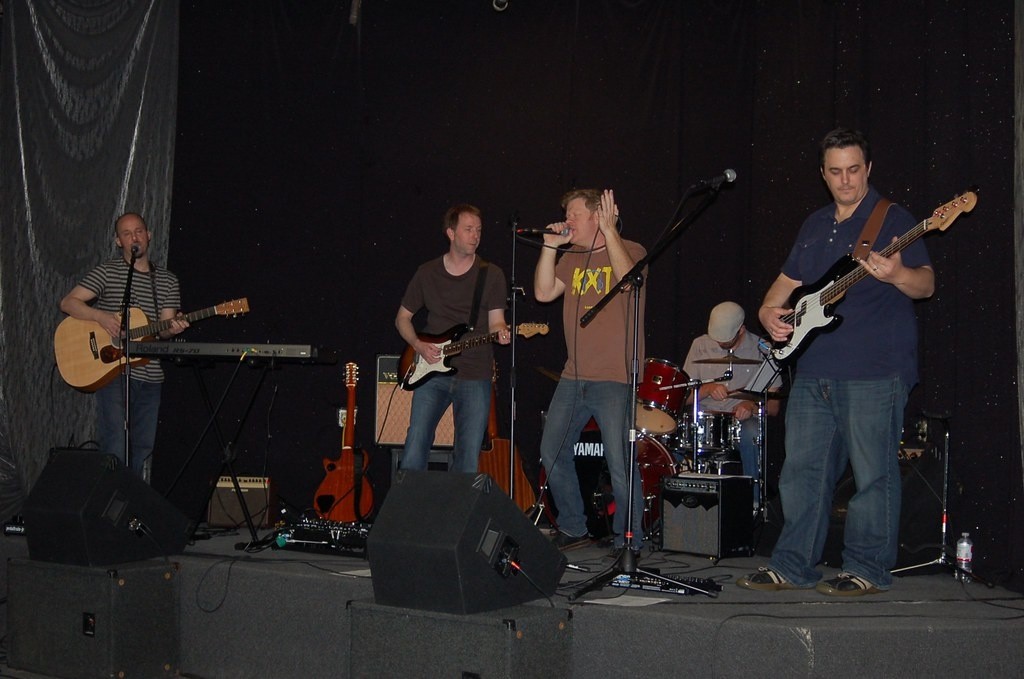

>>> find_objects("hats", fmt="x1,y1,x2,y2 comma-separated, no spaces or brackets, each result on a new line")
707,302,746,342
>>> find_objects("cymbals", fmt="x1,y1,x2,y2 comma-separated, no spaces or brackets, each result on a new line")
727,389,782,401
692,355,763,366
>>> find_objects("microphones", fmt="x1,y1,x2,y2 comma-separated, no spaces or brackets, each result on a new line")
696,169,737,186
131,244,139,253
525,228,569,237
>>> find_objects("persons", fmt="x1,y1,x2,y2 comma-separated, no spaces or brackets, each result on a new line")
534,189,649,559
736,126,935,597
399,203,511,473
683,301,784,514
60,212,189,485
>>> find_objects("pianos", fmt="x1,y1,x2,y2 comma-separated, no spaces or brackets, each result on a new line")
124,338,322,549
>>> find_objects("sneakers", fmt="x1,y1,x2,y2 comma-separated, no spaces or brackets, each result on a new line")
549,532,591,552
738,567,816,589
815,572,887,596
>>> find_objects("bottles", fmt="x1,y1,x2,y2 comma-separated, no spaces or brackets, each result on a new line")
955,533,974,585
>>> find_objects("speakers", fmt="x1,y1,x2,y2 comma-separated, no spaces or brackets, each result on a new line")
366,469,569,616
661,474,754,558
206,477,271,529
756,441,984,577
22,447,197,567
375,354,456,447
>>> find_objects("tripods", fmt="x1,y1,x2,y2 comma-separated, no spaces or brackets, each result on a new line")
569,185,724,600
889,426,996,590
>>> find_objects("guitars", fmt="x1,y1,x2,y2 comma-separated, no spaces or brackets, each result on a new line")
312,360,377,525
396,320,550,391
54,297,251,394
769,189,978,367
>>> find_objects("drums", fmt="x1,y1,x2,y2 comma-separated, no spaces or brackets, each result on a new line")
633,357,691,436
678,409,736,452
536,434,679,546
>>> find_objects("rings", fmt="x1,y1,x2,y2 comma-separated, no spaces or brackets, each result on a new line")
873,267,878,271
502,336,506,339
